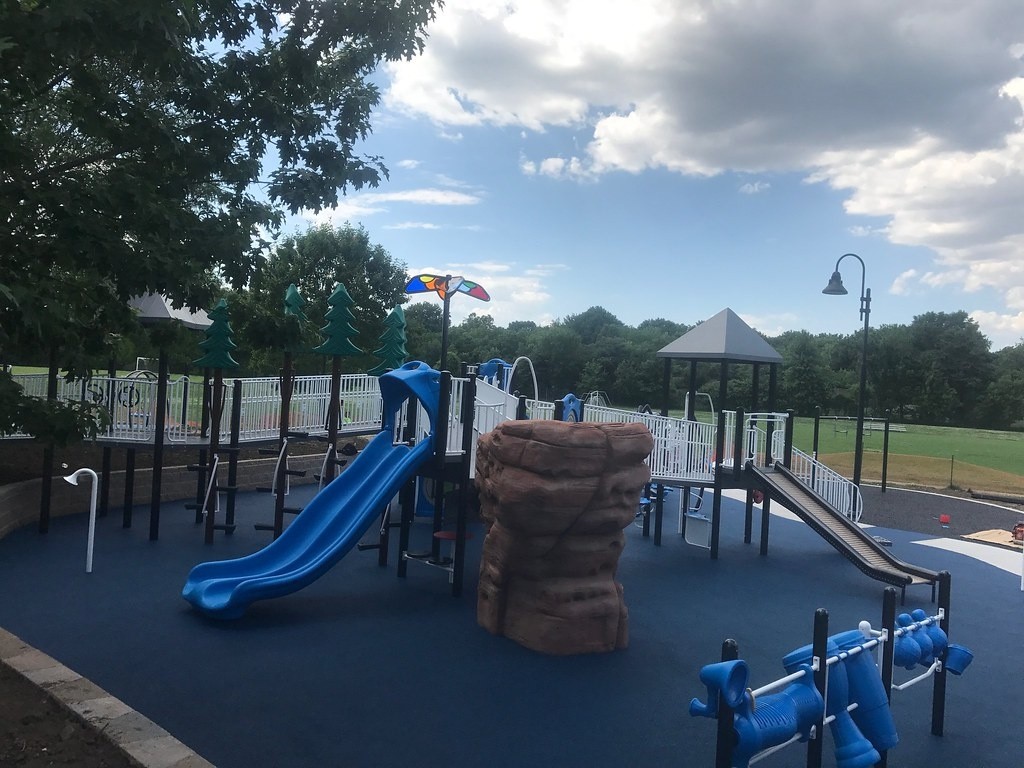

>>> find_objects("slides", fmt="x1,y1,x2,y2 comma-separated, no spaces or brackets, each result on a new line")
744,458,942,588
180,430,434,625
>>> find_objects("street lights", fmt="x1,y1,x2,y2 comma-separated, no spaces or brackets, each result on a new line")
823,254,872,518
